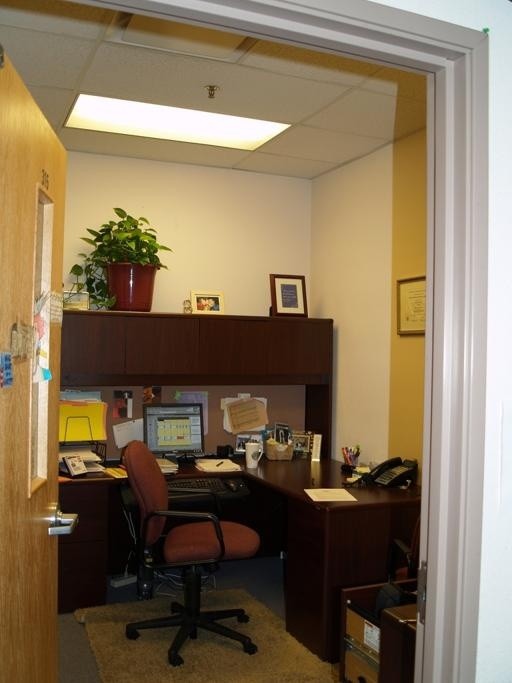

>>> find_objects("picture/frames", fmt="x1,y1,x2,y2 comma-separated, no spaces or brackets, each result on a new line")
269,272,308,318
191,289,225,316
394,274,429,337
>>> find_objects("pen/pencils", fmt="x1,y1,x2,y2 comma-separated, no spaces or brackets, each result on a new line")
215,461,224,467
341,443,361,467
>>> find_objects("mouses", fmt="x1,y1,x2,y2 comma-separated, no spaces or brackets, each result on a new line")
227,478,240,491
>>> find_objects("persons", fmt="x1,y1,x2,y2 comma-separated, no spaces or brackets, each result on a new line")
198,297,220,311
294,439,302,449
238,437,257,451
144,387,157,403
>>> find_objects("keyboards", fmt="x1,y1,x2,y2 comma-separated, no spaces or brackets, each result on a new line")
165,477,220,493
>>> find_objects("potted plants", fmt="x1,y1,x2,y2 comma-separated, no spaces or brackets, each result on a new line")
60,206,175,314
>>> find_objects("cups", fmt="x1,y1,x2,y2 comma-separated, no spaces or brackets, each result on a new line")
244,442,264,469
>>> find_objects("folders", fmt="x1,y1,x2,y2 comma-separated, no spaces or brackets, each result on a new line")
57,391,109,442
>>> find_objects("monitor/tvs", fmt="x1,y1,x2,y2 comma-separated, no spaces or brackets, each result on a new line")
143,402,204,465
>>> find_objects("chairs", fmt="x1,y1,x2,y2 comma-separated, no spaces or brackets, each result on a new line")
119,436,262,667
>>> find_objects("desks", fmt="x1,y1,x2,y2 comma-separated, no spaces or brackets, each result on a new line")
55,452,422,665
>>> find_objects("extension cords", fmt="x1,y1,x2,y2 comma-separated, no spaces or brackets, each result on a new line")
110,575,137,588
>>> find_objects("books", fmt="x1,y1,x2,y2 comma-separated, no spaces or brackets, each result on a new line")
154,459,179,474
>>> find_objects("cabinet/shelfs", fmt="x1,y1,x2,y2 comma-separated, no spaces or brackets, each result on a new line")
60,301,334,390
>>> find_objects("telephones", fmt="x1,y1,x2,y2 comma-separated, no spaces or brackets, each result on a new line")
340,457,417,489
